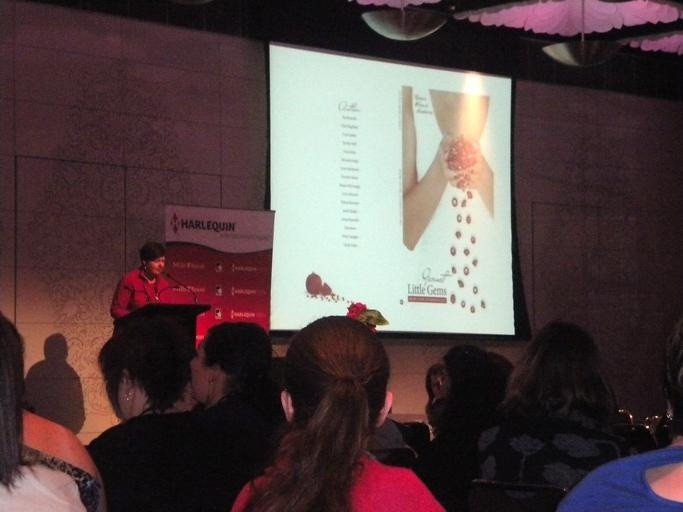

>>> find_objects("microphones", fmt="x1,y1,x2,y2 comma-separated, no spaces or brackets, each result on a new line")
166,273,198,304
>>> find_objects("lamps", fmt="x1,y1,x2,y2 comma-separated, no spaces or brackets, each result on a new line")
533,1,619,71
360,1,448,43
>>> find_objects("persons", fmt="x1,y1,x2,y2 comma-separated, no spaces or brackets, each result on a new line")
402,86,493,250
